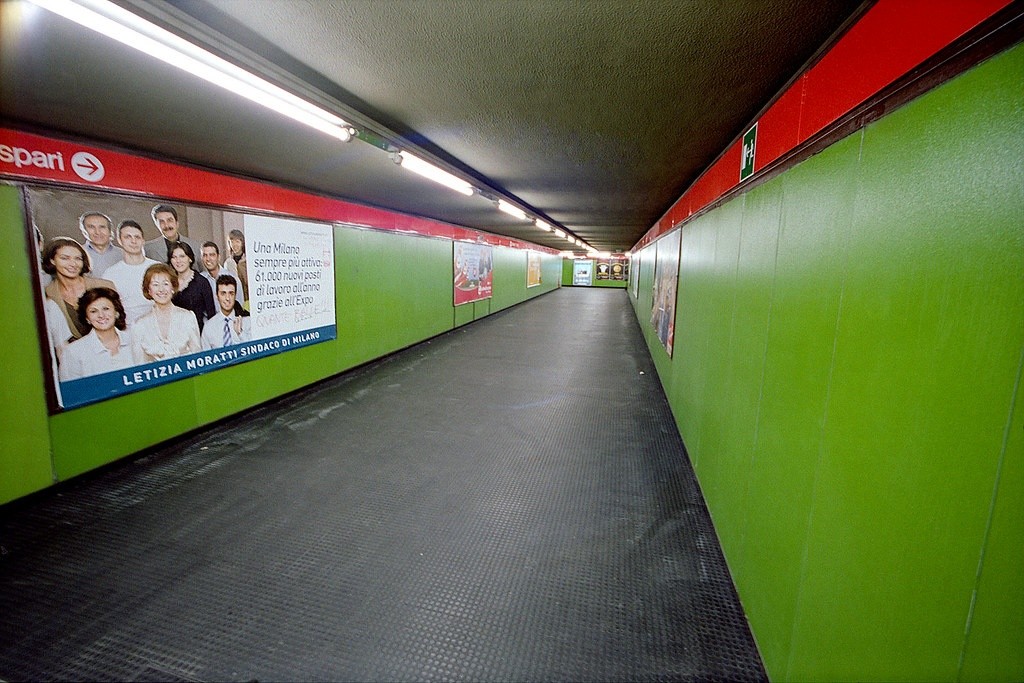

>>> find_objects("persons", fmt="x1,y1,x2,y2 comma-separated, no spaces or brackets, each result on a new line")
59,287,135,382
201,274,252,350
223,227,249,301
42,236,117,338
200,239,245,315
143,204,201,272
104,219,161,321
129,264,202,364
166,241,216,336
80,209,126,279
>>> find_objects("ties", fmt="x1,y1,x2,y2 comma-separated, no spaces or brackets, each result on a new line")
223,318,232,348
